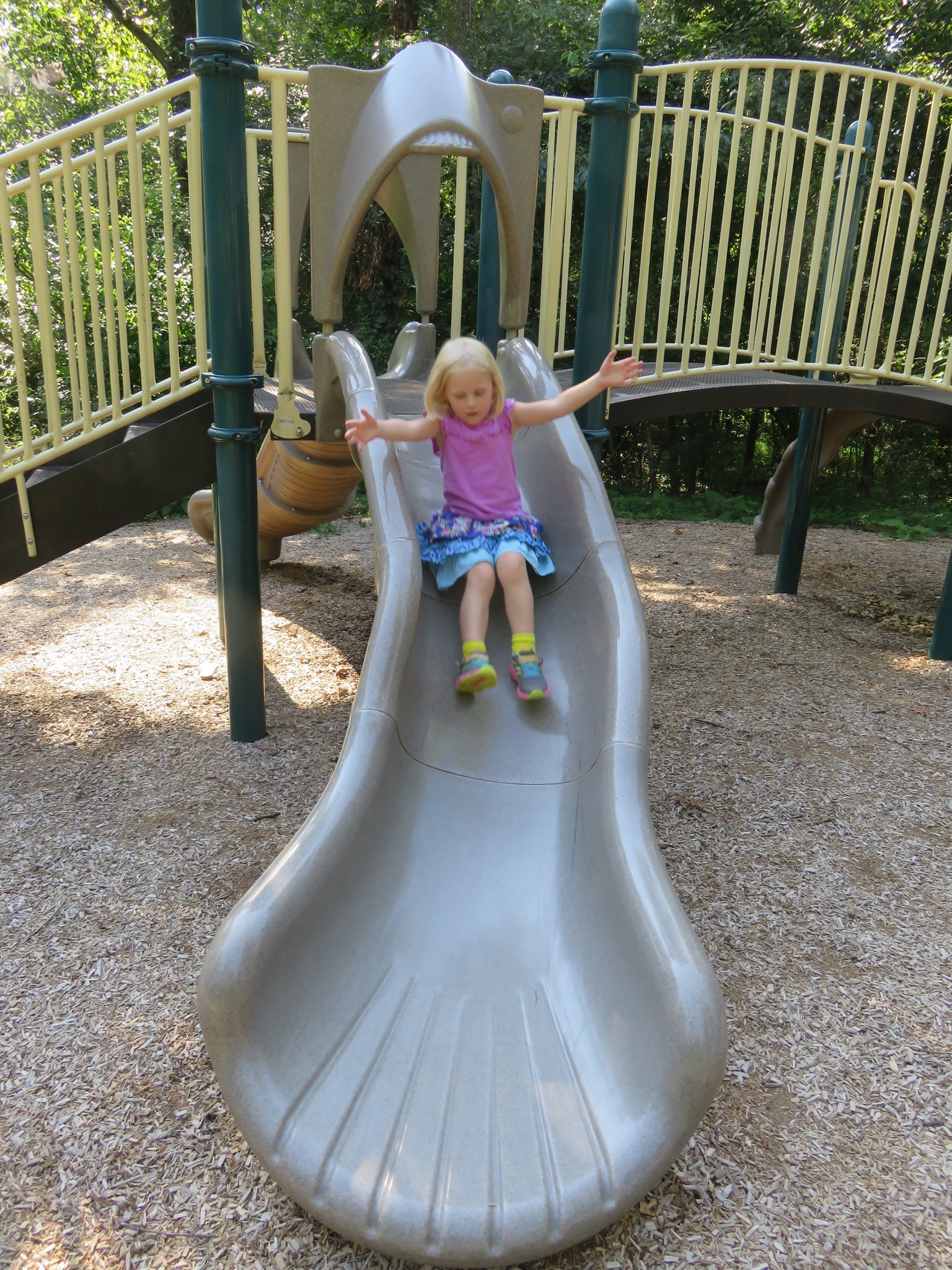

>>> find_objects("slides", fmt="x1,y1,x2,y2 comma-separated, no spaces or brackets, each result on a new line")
200,331,723,1269
188,320,434,561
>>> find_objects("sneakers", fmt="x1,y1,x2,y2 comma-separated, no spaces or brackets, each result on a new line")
455,650,498,692
509,646,549,699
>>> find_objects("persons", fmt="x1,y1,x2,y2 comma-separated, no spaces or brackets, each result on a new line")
342,334,649,707
